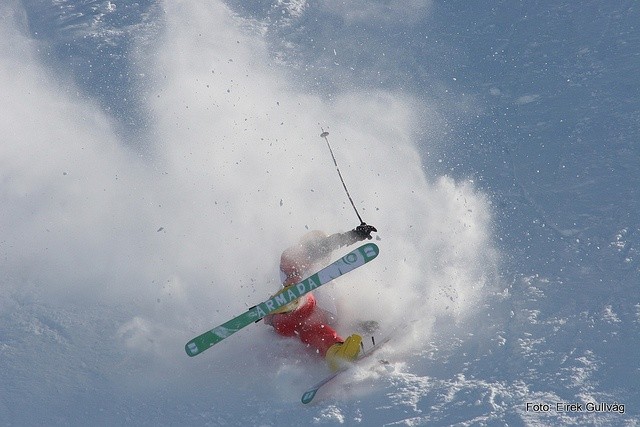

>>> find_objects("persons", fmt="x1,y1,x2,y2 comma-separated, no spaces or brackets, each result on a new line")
265,224,377,367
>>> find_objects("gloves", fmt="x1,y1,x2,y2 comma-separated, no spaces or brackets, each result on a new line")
347,222,376,241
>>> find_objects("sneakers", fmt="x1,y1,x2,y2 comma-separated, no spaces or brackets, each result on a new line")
324,332,366,373
270,285,300,313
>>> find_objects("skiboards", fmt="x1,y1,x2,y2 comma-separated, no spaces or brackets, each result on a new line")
184,241,416,404
